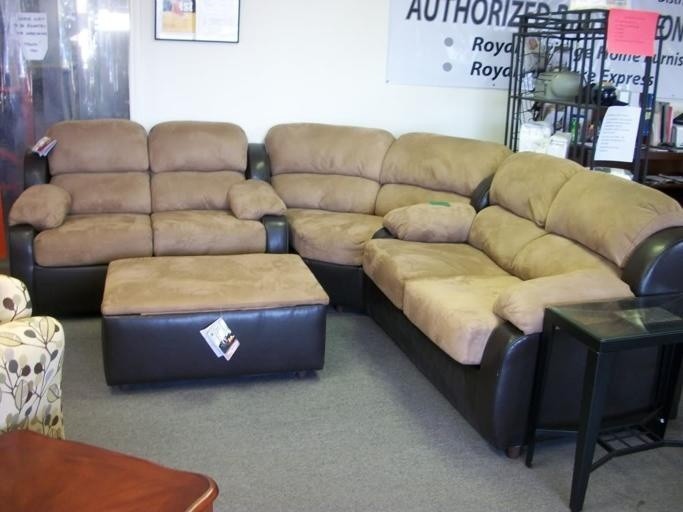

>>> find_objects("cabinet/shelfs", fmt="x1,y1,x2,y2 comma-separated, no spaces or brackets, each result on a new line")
503,8,667,186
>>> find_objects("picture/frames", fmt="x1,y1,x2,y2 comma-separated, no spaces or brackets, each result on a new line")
154,0,242,42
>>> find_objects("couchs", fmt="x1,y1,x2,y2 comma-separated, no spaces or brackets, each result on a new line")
5,117,289,324
267,122,682,464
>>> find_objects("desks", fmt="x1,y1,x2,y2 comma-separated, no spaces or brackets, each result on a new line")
0,428,219,511
525,301,682,511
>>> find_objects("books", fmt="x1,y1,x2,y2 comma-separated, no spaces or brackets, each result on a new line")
641,92,673,148
